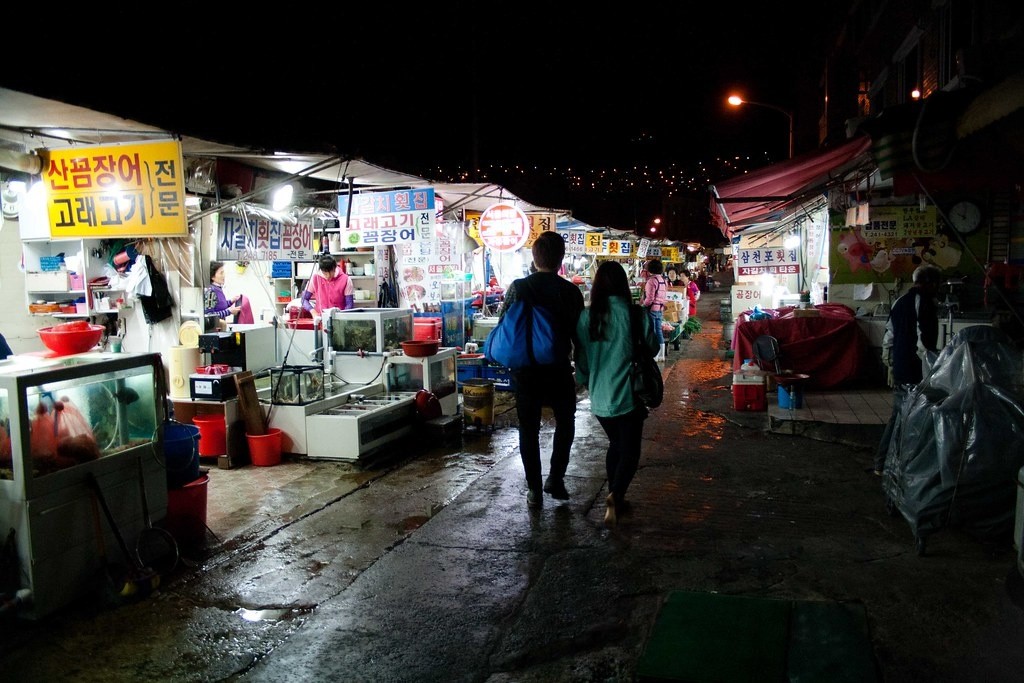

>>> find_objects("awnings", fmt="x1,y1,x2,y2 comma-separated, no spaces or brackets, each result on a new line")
0,88,691,259
708,134,874,243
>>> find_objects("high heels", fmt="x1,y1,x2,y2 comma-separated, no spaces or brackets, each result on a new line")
603,491,620,530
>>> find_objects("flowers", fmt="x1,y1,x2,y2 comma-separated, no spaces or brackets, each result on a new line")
800,290,810,302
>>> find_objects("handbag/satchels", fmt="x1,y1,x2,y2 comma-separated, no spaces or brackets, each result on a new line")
484,298,569,369
628,304,663,408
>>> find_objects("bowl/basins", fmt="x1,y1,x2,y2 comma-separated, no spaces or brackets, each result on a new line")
352,268,364,276
770,372,810,383
399,340,440,357
415,389,442,421
35,325,106,355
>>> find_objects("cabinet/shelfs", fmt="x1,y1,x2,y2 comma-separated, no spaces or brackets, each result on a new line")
272,260,318,316
313,228,377,309
21,239,125,318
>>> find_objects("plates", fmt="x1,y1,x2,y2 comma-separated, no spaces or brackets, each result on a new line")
356,248,373,251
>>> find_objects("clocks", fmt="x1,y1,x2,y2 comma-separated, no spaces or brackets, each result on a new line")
948,200,985,235
0,174,19,219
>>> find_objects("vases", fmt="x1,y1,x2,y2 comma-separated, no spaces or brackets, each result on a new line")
800,302,810,309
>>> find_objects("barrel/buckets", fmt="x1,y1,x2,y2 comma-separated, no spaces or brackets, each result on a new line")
461,378,495,427
163,424,200,487
192,413,226,458
718,350,727,360
777,382,803,409
723,322,734,340
168,474,210,536
245,428,282,467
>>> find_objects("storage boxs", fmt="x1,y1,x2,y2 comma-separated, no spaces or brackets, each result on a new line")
457,354,513,391
440,281,471,301
732,370,767,410
663,286,689,338
271,366,325,406
414,317,442,346
383,347,458,415
322,308,414,356
0,352,165,500
794,308,820,318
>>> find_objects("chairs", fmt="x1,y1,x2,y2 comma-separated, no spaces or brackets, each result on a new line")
753,335,781,375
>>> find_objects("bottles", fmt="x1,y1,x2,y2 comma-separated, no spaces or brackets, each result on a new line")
340,257,353,276
742,359,760,371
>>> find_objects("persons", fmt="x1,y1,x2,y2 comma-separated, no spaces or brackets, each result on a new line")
667,266,700,317
873,265,942,476
499,231,585,506
641,258,668,362
0,333,13,361
301,251,354,330
204,261,241,329
575,261,660,522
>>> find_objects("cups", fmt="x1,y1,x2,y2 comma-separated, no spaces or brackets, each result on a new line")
329,241,340,252
109,336,121,352
365,264,374,275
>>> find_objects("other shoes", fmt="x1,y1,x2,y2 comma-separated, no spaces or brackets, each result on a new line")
544,478,571,500
527,489,544,508
